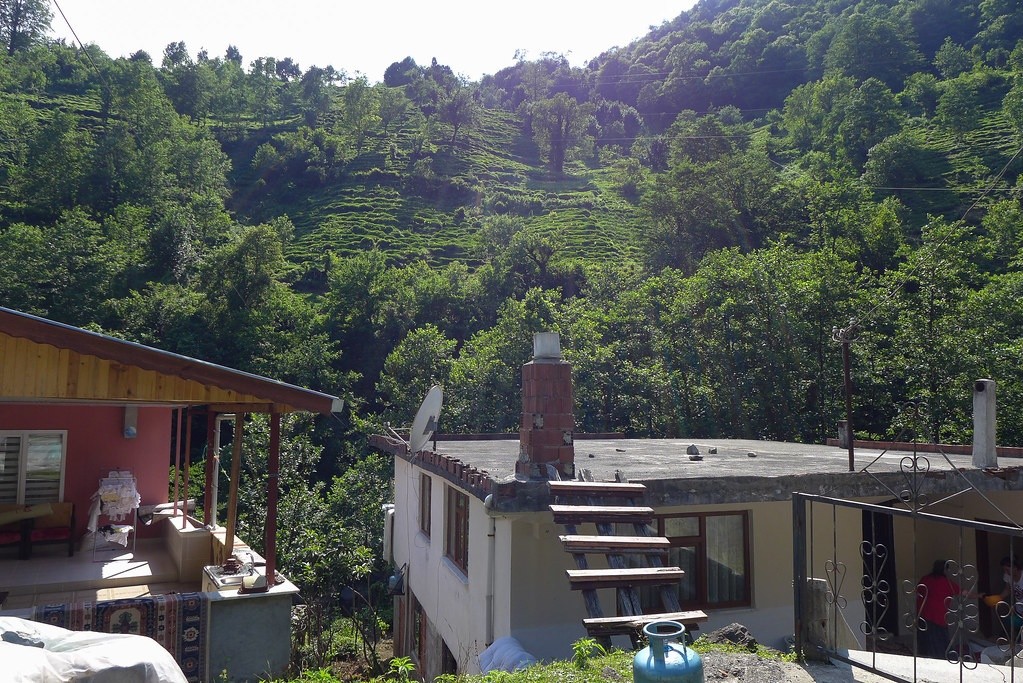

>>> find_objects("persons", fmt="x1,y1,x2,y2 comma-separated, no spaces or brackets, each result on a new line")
993,555,1023,643
914,560,986,660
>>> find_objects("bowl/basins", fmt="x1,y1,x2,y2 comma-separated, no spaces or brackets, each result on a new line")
985,595,1002,606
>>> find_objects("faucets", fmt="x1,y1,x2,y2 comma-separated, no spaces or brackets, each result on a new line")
242,552,254,576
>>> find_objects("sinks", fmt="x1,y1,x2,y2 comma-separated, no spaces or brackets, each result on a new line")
217,574,244,586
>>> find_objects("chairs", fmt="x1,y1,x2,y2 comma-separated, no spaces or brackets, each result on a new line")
92,478,137,563
0,502,75,560
